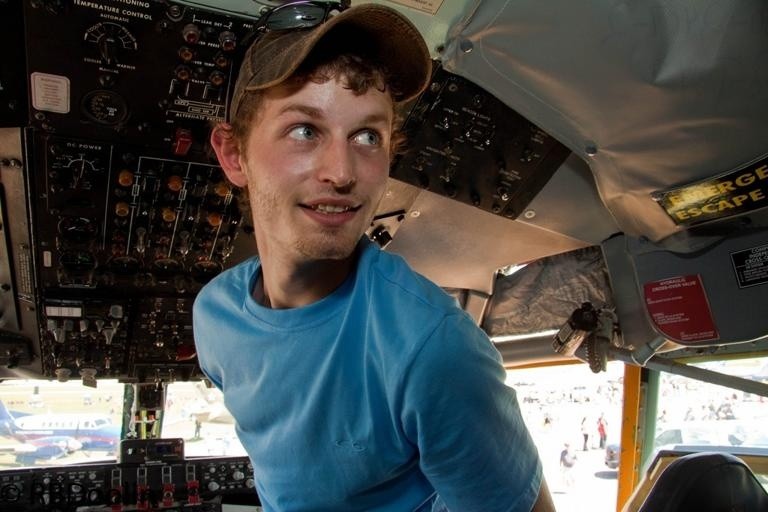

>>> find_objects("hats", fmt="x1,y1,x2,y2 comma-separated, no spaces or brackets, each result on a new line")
225,3,433,124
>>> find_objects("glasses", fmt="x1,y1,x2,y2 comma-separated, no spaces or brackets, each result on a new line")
226,0,351,124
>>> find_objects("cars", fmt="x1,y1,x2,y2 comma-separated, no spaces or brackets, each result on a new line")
605,422,741,472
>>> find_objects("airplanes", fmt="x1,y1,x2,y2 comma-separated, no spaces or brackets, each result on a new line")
0,398,122,465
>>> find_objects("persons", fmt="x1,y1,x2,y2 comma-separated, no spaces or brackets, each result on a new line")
542,374,768,471
190,5,559,511
